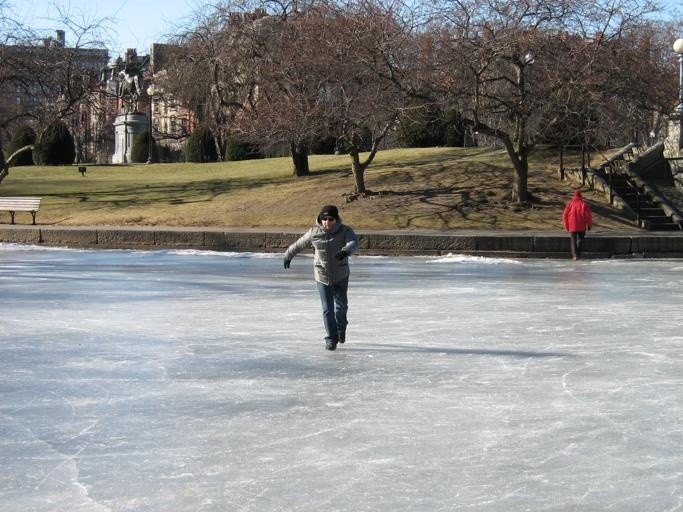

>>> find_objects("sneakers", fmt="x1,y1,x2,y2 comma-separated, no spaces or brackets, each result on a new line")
325,341,338,350
338,330,345,343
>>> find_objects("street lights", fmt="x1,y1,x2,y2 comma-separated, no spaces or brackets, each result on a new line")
526,54,535,128
147,88,154,163
673,38,683,103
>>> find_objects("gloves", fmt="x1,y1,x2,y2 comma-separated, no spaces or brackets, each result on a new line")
284,256,291,269
335,251,349,261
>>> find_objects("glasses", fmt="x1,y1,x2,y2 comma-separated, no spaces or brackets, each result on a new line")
321,218,335,221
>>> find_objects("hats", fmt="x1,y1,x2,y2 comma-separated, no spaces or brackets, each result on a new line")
319,206,339,221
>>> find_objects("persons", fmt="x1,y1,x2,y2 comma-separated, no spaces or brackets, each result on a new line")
562,190,592,260
283,205,359,350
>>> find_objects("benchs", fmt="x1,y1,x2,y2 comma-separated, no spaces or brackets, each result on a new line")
0,197,42,224
